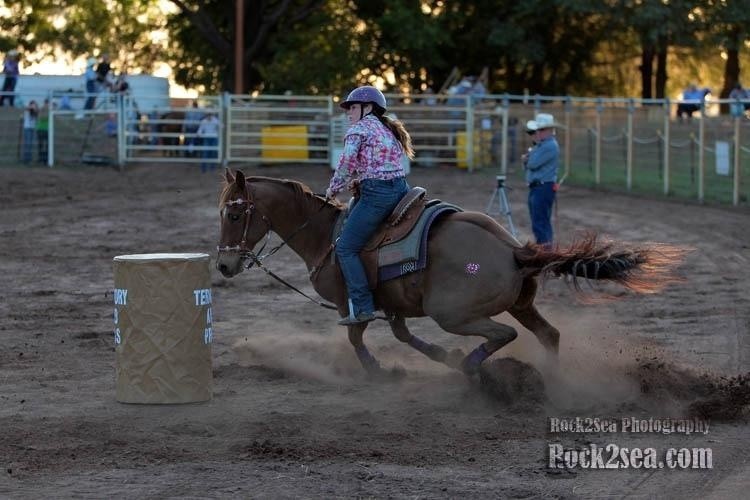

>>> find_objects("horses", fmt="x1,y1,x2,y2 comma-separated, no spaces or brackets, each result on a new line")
676,88,711,120
155,111,185,157
215,166,696,394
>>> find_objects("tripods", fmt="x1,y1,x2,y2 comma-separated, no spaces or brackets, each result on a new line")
485,176,516,235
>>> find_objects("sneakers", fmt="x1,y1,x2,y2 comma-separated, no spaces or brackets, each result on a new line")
337,310,375,326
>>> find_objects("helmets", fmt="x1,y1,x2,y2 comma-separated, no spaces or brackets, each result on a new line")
340,86,387,115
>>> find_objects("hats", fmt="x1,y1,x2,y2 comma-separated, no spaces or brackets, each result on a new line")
527,114,567,131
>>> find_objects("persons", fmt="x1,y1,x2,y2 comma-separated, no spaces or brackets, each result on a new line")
321,88,408,325
519,114,561,249
0,51,223,174
674,84,698,117
727,82,749,116
